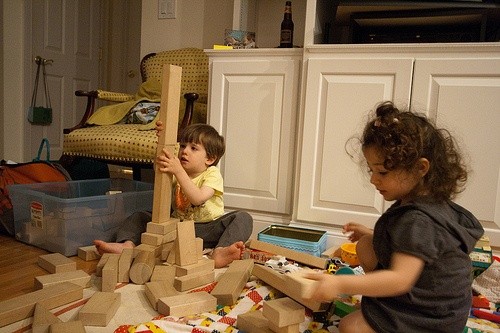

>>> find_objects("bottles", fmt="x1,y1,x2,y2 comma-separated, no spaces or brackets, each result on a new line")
279,1,294,48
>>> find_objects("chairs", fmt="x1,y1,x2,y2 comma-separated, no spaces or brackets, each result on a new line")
63,47,210,180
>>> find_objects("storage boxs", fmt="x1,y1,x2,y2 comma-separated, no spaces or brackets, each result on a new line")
8,179,154,257
257,224,327,257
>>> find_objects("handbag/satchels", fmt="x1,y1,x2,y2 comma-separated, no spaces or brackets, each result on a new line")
0,161,71,238
29,106,52,124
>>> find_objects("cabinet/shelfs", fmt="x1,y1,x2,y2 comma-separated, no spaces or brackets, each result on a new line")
203,48,301,224
299,43,500,252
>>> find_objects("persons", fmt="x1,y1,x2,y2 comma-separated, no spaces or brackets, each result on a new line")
93,120,253,270
302,101,485,333
